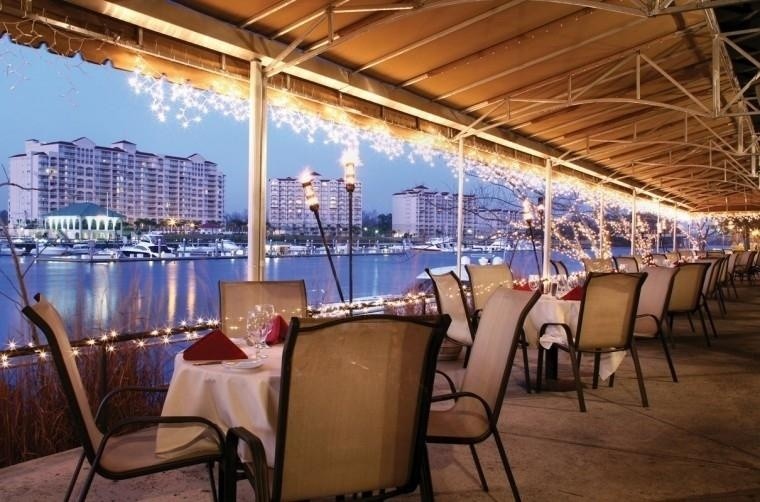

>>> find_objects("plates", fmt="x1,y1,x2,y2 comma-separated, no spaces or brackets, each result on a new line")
222,358,263,370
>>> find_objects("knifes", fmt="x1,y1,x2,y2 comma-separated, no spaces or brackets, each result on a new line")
193,361,222,366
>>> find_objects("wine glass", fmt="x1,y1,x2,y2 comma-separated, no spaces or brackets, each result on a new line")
528,277,559,296
254,304,275,349
246,309,270,359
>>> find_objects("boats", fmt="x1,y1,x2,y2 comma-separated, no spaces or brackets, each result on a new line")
423,236,552,256
63,234,410,260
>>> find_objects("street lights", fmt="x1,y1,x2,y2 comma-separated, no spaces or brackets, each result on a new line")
340,160,357,301
294,167,347,317
